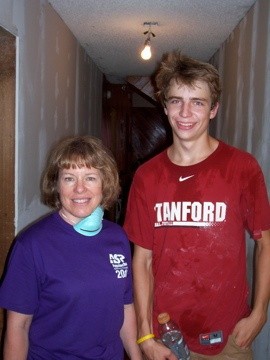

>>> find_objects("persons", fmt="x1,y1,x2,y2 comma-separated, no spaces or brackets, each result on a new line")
123,49,270,360
0,135,145,360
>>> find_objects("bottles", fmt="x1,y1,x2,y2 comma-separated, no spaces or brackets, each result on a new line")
157,312,191,360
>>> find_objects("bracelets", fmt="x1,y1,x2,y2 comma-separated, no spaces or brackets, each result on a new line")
137,334,154,344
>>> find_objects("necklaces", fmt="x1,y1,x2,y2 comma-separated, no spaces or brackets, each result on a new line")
61,211,77,224
